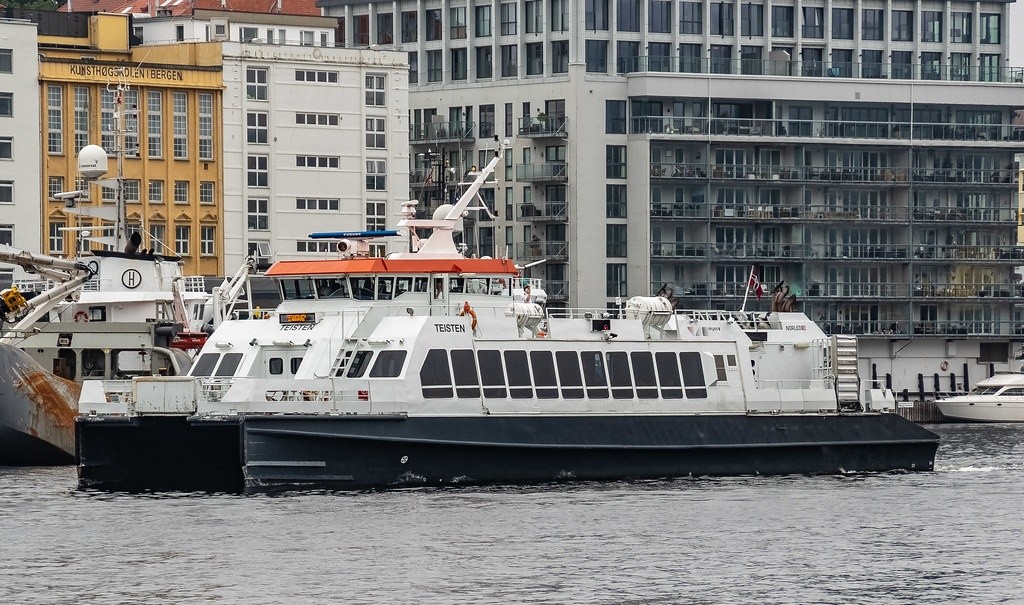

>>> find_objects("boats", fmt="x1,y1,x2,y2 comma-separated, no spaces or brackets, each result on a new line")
933,373,1024,422
0,137,939,485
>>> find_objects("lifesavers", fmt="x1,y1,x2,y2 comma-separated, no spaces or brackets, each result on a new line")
941,361,949,371
74,311,89,323
461,309,478,329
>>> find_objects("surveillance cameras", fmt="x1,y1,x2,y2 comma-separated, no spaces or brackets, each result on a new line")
606,333,617,342
154,255,163,262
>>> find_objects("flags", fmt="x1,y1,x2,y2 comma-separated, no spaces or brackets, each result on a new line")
749,273,763,298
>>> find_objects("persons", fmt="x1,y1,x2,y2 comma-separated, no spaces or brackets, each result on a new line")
522,285,532,303
